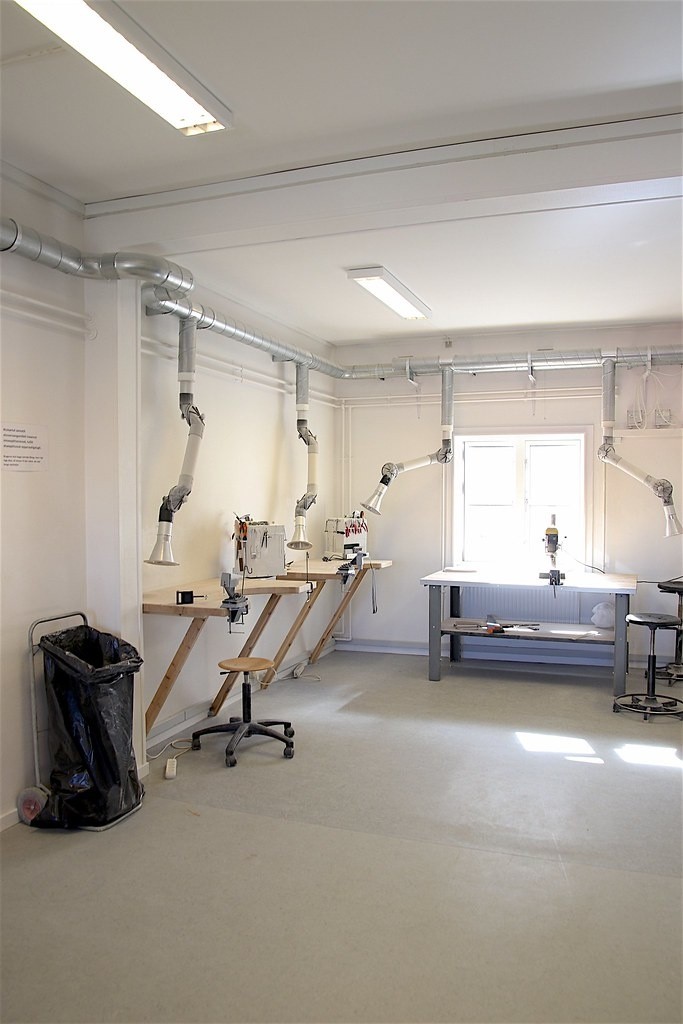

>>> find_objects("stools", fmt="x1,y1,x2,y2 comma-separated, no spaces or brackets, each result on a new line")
191,657,295,767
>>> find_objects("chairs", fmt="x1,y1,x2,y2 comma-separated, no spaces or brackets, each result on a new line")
645,581,683,686
614,613,683,721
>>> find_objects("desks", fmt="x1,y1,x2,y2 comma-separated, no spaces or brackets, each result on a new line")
262,560,392,691
143,576,316,736
420,564,636,697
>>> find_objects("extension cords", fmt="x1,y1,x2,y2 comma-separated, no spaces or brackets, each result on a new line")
165,759,177,778
293,665,304,677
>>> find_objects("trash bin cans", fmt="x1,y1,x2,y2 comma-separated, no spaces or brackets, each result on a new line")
30,625,145,828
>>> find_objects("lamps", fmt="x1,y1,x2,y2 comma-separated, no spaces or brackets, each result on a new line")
347,267,431,319
14,0,234,139
